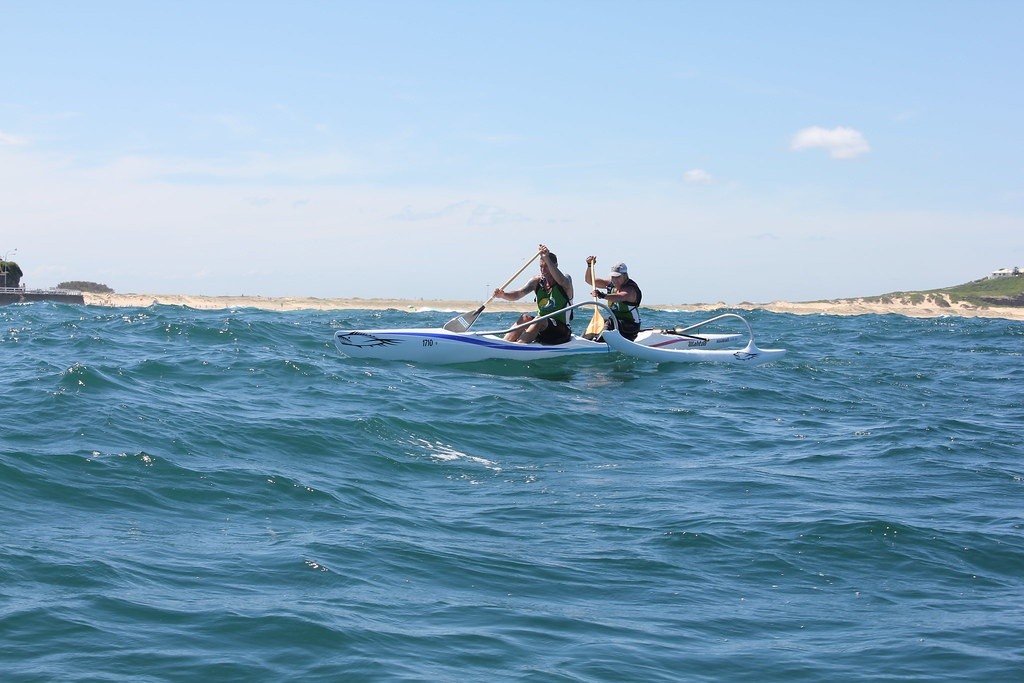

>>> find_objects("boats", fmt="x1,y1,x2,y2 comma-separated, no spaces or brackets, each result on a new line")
334,301,786,365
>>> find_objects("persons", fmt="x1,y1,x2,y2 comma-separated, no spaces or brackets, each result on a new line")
493,244,574,344
584,255,642,343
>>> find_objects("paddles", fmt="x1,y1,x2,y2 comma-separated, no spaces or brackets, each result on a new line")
585,256,605,340
444,244,545,333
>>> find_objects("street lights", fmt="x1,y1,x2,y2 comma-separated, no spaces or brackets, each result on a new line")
4,247,18,293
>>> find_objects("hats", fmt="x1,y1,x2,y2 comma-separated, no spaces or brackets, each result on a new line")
610,261,627,277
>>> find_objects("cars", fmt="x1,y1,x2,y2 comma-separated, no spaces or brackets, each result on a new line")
48,286,56,295
